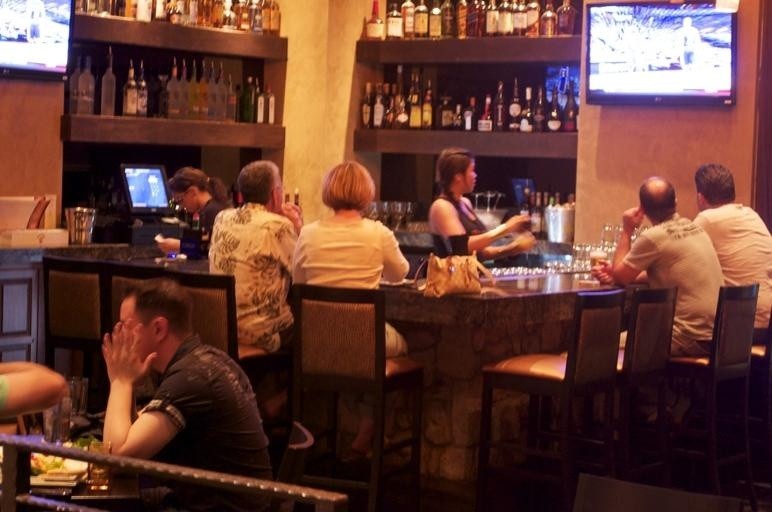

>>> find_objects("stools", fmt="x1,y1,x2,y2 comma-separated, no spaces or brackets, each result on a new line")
653,284,762,510
475,285,630,509
614,286,679,491
40,257,287,456
743,319,771,485
282,279,426,511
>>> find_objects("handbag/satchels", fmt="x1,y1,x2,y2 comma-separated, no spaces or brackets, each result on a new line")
414,253,497,295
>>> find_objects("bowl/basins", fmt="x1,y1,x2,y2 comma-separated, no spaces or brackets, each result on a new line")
475,209,508,229
546,209,575,243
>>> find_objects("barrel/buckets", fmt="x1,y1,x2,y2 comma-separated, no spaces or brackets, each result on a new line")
543,207,576,244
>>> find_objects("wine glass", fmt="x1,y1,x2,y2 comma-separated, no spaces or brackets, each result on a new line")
364,201,417,231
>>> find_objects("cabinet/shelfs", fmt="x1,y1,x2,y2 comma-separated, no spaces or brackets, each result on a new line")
348,26,581,161
58,9,289,152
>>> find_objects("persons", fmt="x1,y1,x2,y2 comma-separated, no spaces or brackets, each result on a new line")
692,162,772,348
428,147,537,272
290,161,416,462
99,278,274,512
0,361,70,420
155,166,228,260
206,160,304,421
591,176,725,440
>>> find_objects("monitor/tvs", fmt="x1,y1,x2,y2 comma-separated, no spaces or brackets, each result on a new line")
0,0,78,82
584,3,738,108
120,163,173,213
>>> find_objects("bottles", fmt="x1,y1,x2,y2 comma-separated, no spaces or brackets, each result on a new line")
75,0,280,34
78,50,274,125
360,2,581,135
520,188,575,234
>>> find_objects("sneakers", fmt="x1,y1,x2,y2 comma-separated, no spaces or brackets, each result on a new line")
286,421,316,453
350,430,391,461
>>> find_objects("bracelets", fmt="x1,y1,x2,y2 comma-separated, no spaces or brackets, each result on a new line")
495,241,517,258
486,224,509,240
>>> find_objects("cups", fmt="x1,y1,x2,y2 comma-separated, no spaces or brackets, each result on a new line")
63,376,88,415
471,192,507,208
573,226,624,267
88,443,113,492
41,406,71,440
